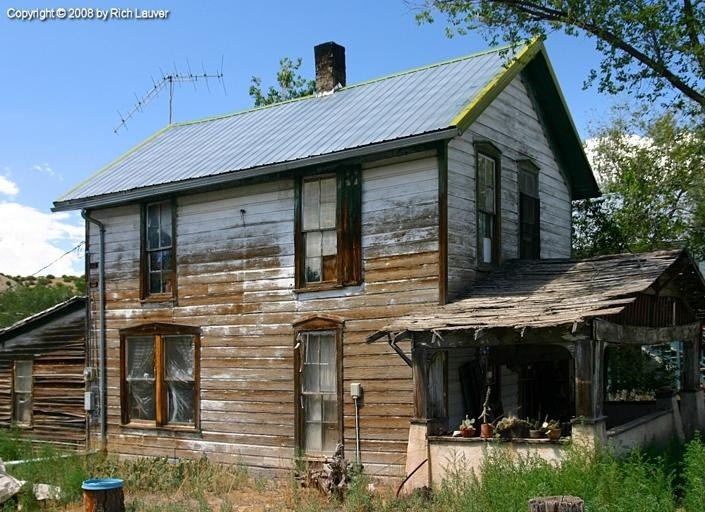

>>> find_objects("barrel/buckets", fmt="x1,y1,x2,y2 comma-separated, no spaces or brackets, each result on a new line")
81,478,124,512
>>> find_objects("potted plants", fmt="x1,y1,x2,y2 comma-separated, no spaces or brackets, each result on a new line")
460,415,476,437
478,386,491,438
527,413,548,439
546,420,560,439
